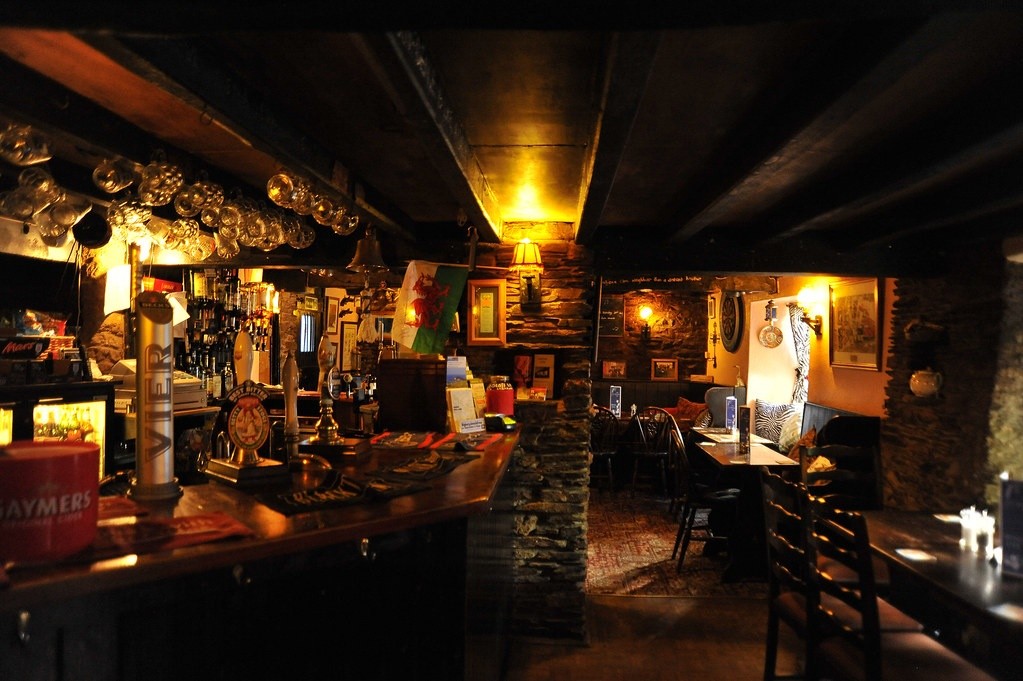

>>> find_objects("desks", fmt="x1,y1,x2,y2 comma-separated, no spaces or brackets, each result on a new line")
600,410,691,479
686,424,774,471
817,510,1023,681
694,441,800,575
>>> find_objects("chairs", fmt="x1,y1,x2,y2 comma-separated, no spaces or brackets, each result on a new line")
589,385,757,569
760,466,992,681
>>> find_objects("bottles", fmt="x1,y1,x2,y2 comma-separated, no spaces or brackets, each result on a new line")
960,508,995,559
170,268,270,399
486,377,514,416
631,404,637,417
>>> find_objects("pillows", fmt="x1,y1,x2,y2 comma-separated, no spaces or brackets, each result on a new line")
674,396,713,427
787,426,817,464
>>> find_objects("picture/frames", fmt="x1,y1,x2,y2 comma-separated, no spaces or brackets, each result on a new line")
602,359,627,380
651,359,678,382
829,277,881,372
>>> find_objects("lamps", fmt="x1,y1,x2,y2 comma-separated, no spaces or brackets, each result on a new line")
797,289,823,336
640,308,652,341
508,243,543,312
